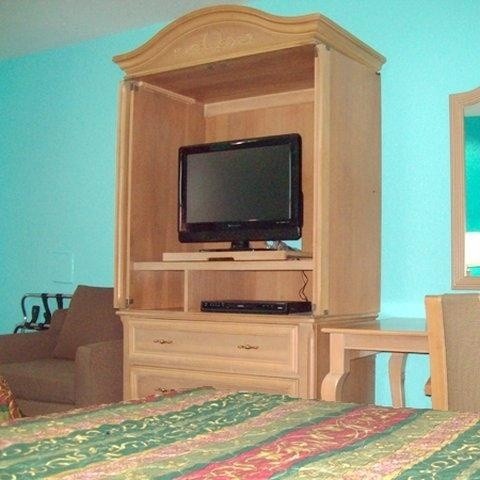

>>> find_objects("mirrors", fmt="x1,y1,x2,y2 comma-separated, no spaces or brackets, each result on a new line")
449,86,479,291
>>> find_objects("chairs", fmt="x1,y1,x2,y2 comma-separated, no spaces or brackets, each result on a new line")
0,286,123,415
426,293,479,412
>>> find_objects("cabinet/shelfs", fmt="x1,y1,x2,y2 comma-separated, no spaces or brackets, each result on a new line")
112,5,385,405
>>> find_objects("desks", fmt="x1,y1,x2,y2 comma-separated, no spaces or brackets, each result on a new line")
320,320,431,409
1,386,479,479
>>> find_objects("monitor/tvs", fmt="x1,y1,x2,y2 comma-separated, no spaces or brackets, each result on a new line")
177,132,304,253
